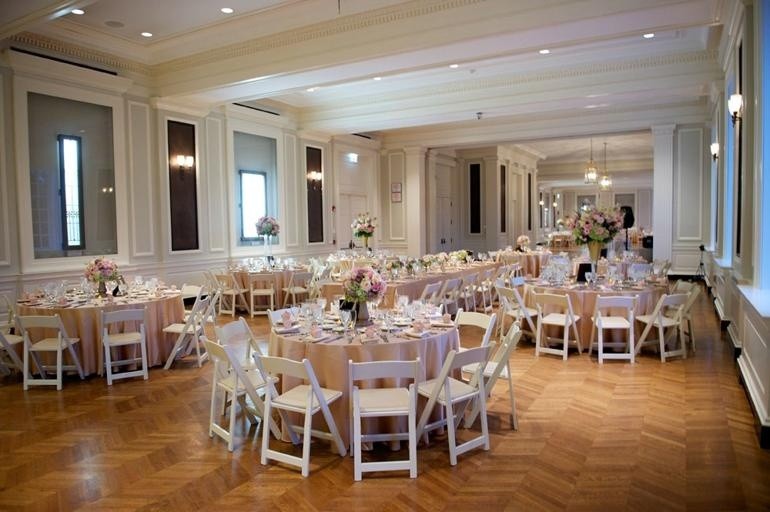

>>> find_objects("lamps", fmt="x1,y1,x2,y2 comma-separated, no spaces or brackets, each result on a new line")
583,136,600,185
727,94,743,129
709,140,719,162
305,170,322,192
597,142,614,192
176,154,194,181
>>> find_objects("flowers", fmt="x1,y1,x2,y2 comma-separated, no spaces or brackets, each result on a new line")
567,197,626,245
350,209,380,239
254,216,283,237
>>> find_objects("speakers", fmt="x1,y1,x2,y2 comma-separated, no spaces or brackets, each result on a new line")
642,236,653,248
620,206,634,229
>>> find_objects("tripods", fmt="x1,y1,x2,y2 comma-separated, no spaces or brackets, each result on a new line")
696,251,706,279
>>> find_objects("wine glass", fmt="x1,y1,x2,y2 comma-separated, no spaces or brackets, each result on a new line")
18,274,159,307
281,293,450,342
333,247,486,287
540,254,650,291
230,258,296,271
